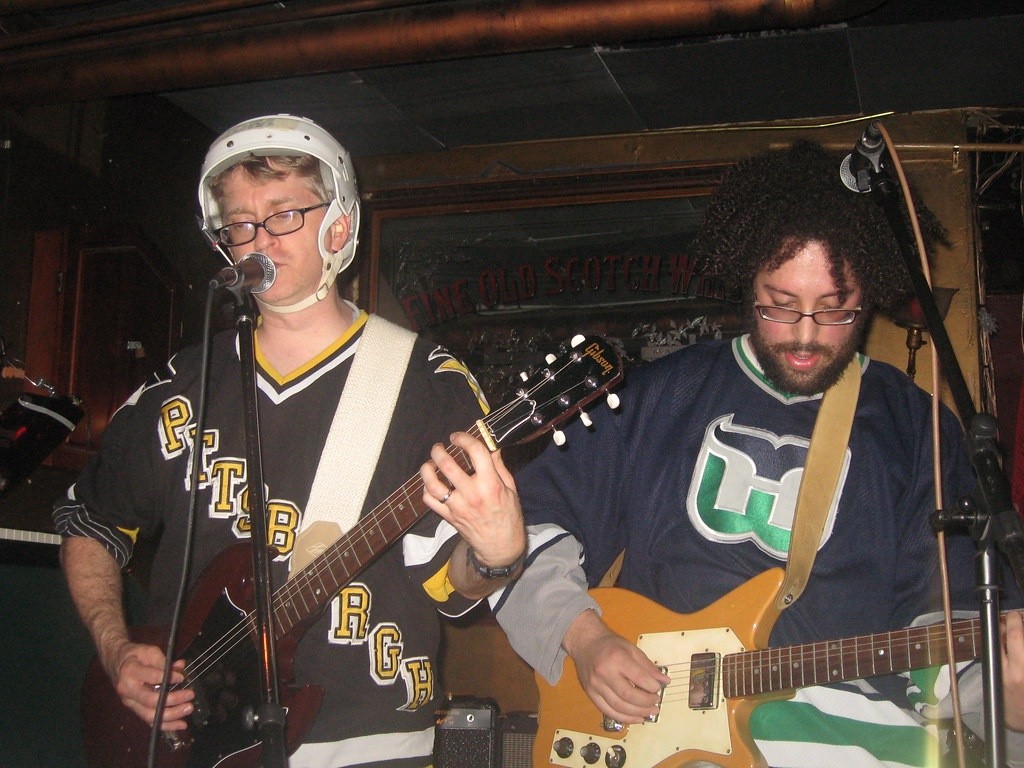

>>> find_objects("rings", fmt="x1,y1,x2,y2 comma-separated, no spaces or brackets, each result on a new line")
439,488,453,504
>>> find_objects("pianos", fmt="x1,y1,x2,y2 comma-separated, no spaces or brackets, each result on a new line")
0,398,131,570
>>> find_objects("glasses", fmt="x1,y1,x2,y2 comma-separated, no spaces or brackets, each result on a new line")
750,281,864,326
212,202,331,247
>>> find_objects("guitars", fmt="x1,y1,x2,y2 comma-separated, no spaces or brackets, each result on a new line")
78,329,629,768
530,569,1024,768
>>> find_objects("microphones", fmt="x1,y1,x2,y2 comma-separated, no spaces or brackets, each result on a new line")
209,252,278,294
839,119,886,194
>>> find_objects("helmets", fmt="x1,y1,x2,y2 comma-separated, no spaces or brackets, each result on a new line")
197,112,362,275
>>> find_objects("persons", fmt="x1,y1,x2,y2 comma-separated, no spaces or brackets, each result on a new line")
49,113,529,767
485,144,1024,768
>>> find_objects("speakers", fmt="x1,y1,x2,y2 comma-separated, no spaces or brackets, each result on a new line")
434,693,503,768
